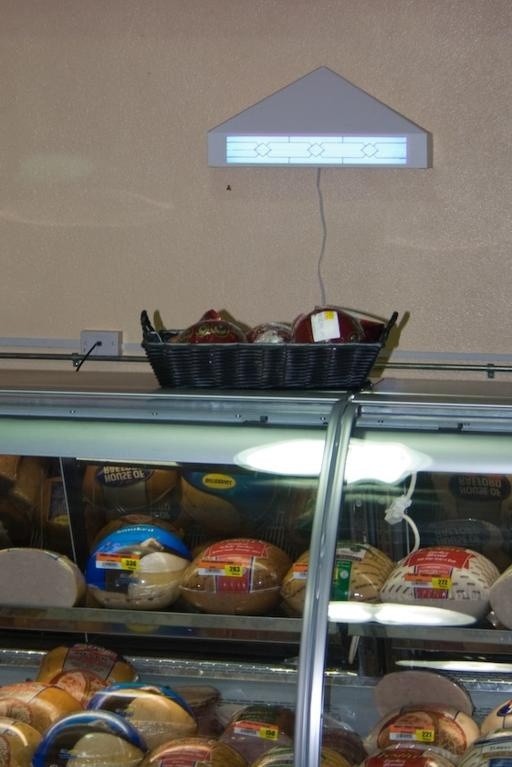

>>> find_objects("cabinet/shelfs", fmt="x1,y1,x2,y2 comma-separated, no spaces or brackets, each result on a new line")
1,366,510,766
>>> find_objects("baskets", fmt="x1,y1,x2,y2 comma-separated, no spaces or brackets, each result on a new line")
138,305,402,394
